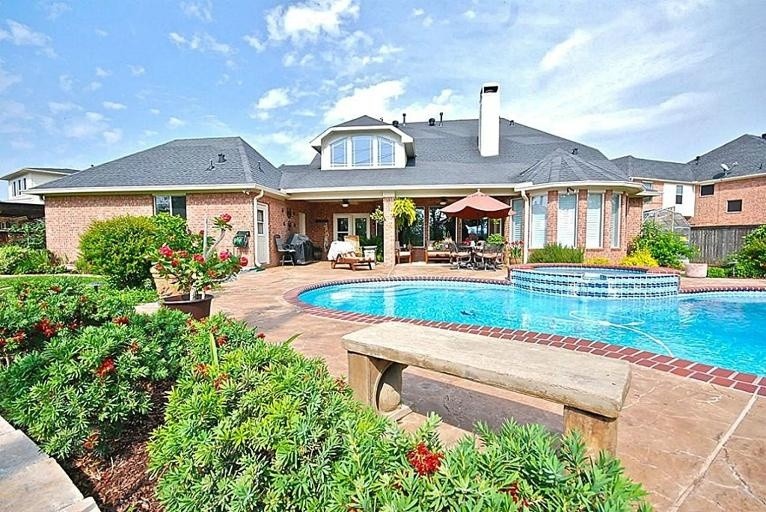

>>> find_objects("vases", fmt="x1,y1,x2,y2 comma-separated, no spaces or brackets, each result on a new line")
509,257,522,264
161,294,212,321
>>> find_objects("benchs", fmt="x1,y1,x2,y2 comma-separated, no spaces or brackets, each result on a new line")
341,322,630,465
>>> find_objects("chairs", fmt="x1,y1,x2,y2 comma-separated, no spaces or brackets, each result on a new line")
330,241,374,271
448,240,508,271
395,241,411,265
274,234,296,266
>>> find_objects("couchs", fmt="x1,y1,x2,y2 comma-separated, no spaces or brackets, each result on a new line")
424,240,449,264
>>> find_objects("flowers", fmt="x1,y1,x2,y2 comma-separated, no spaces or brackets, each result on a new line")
509,240,525,258
155,215,248,299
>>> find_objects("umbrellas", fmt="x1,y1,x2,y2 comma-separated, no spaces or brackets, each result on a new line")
439,187,517,265
